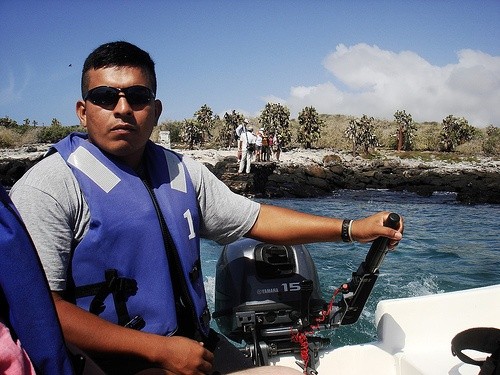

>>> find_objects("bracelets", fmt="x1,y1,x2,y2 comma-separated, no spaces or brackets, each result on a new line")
341,218,359,244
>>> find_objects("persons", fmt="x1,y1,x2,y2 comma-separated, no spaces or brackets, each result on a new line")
238,127,255,174
8,41,403,375
236,119,285,162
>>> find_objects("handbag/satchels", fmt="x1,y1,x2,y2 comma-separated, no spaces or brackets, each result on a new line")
246,143,255,152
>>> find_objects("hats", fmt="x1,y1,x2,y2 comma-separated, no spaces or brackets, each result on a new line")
247,127,253,131
244,119,249,124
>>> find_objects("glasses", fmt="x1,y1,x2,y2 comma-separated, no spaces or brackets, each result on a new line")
84,85,156,106
